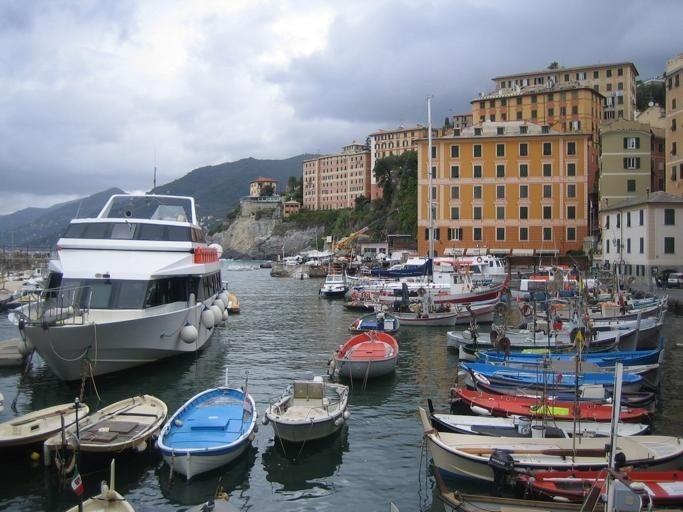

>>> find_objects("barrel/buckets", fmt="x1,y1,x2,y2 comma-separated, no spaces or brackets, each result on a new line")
531,424,546,437
518,416,531,436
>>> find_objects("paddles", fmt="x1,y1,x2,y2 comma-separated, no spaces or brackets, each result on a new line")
11,407,78,426
457,448,606,457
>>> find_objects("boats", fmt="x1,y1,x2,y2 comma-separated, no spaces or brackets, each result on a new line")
451,384,653,425
7,192,230,385
330,329,399,379
489,450,681,504
440,263,670,390
154,369,258,482
425,397,650,442
267,378,351,445
65,458,134,512
267,98,507,325
1,397,89,450
47,394,170,486
414,405,681,478
347,305,402,337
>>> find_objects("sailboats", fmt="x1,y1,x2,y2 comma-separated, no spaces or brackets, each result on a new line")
435,361,680,512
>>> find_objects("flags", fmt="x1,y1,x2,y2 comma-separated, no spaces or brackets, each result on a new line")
70,466,85,497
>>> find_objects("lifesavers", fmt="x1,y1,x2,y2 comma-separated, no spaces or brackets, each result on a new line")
196,246,218,263
